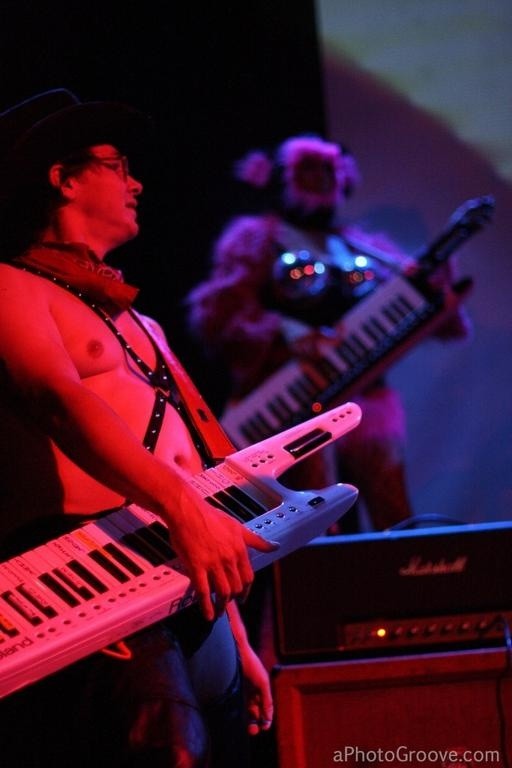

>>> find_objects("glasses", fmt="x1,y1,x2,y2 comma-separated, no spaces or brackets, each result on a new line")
69,155,130,182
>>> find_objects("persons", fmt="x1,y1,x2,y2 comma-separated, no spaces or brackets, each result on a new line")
1,129,279,768
184,133,472,538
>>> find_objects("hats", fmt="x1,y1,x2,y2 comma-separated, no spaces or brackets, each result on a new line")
1,88,160,197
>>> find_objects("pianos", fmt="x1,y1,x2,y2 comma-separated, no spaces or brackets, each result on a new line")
0,401,364,700
222,192,494,453
